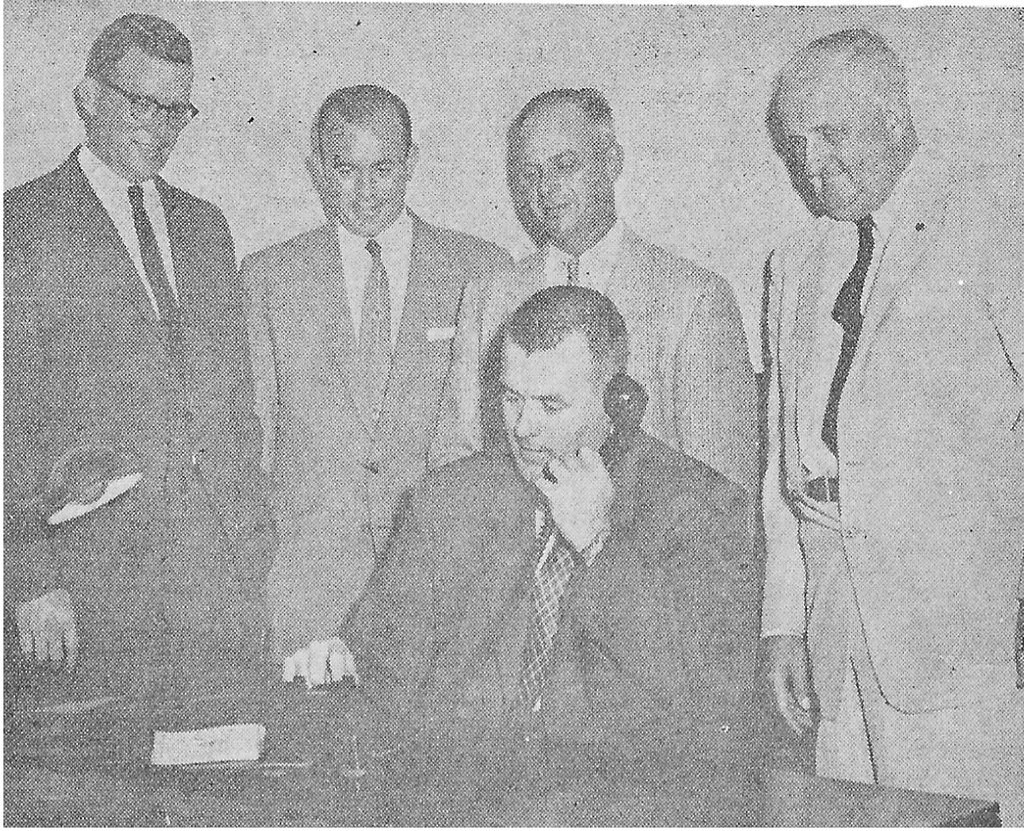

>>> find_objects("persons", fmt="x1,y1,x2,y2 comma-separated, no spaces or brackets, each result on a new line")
231,81,516,680
1,10,241,677
752,24,1024,831
277,283,759,829
428,87,760,489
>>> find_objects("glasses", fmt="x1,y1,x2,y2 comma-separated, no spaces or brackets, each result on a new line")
99,75,199,130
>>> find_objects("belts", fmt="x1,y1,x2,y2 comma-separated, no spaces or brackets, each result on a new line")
804,477,840,504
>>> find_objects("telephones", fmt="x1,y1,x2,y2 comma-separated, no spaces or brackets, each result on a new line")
541,375,649,484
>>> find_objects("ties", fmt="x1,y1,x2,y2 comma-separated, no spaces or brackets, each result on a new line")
821,213,878,460
357,238,393,427
566,256,579,290
507,526,573,711
128,185,194,497
